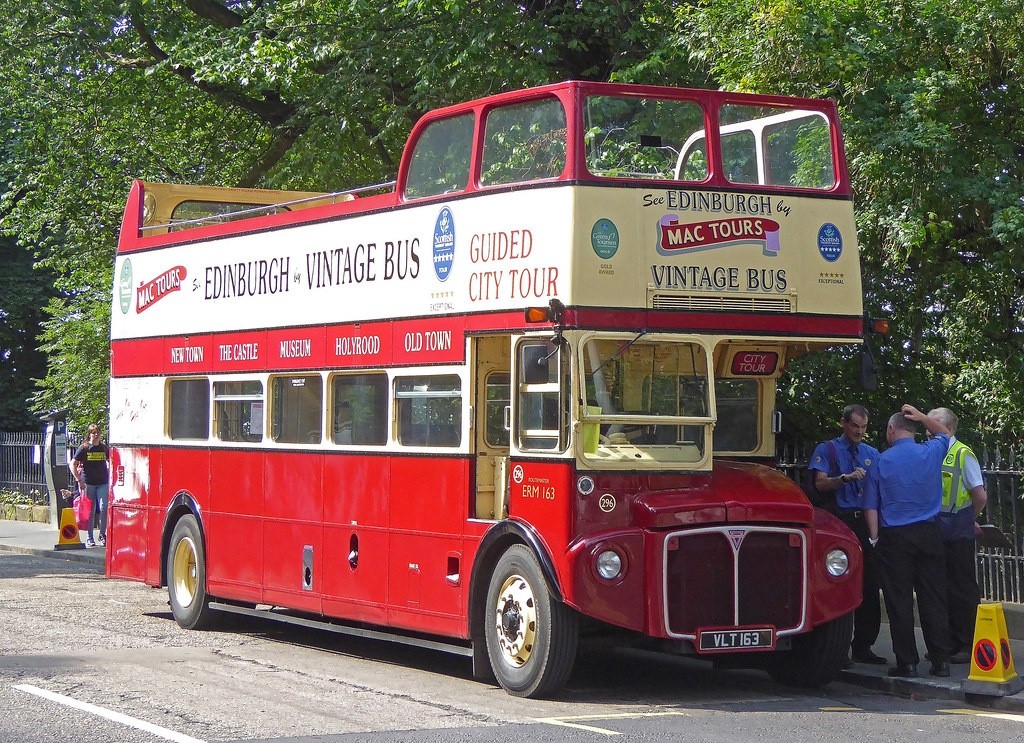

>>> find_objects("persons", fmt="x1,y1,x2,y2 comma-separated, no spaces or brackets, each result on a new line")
925,407,986,664
811,404,887,668
60,424,109,547
863,404,950,677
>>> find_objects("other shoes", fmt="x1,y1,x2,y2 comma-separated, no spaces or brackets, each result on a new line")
853,652,888,663
99,534,106,544
88,539,95,546
840,659,855,669
951,650,970,663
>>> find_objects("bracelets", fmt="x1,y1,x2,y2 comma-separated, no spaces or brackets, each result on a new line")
841,474,847,484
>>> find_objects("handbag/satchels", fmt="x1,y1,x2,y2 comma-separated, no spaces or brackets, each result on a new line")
805,441,838,505
72,488,93,530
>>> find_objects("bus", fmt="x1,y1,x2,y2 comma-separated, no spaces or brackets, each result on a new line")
101,78,891,700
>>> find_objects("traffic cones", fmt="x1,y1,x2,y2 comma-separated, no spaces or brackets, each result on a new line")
53,508,86,550
963,602,1024,697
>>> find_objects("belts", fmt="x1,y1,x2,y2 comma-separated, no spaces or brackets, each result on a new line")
840,510,865,518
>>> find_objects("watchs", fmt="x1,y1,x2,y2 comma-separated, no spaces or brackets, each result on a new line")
868,538,879,544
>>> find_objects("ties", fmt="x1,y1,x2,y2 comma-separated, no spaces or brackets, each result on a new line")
851,446,863,494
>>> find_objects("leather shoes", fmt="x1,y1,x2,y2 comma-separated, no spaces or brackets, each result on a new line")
888,663,918,676
929,662,950,676
